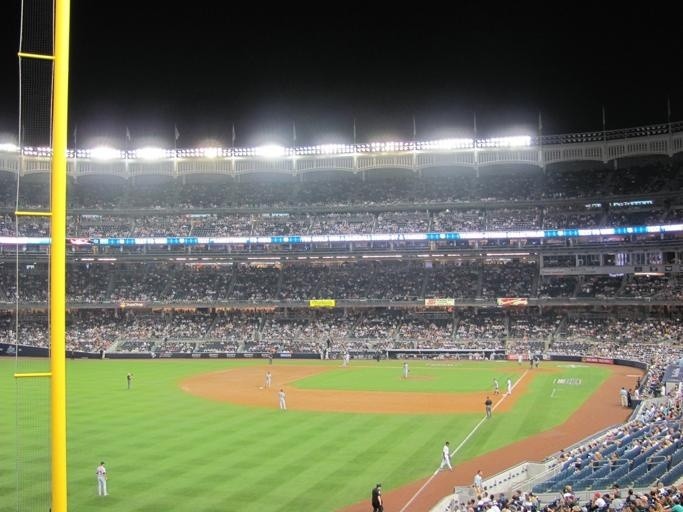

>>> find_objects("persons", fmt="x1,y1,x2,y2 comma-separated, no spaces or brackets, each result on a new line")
265,371,271,387
441,441,452,470
279,389,286,409
453,379,683,512
371,483,382,512
2,168,683,377
127,373,131,388
95,462,107,495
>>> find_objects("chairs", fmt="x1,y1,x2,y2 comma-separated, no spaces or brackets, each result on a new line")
1,303,681,362
430,405,683,512
0,161,683,240
1,228,680,307
